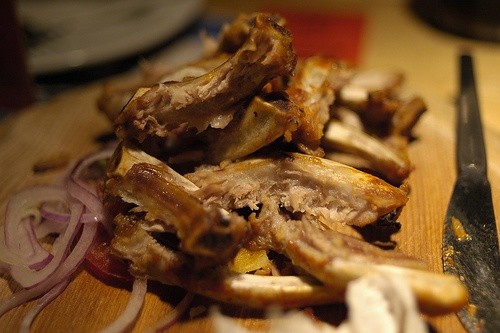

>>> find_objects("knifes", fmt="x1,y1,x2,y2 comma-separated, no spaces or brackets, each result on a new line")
439,45,500,333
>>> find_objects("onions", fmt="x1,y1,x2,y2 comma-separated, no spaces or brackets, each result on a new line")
0,140,195,332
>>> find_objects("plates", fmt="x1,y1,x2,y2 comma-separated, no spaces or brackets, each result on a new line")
0,0,208,76
